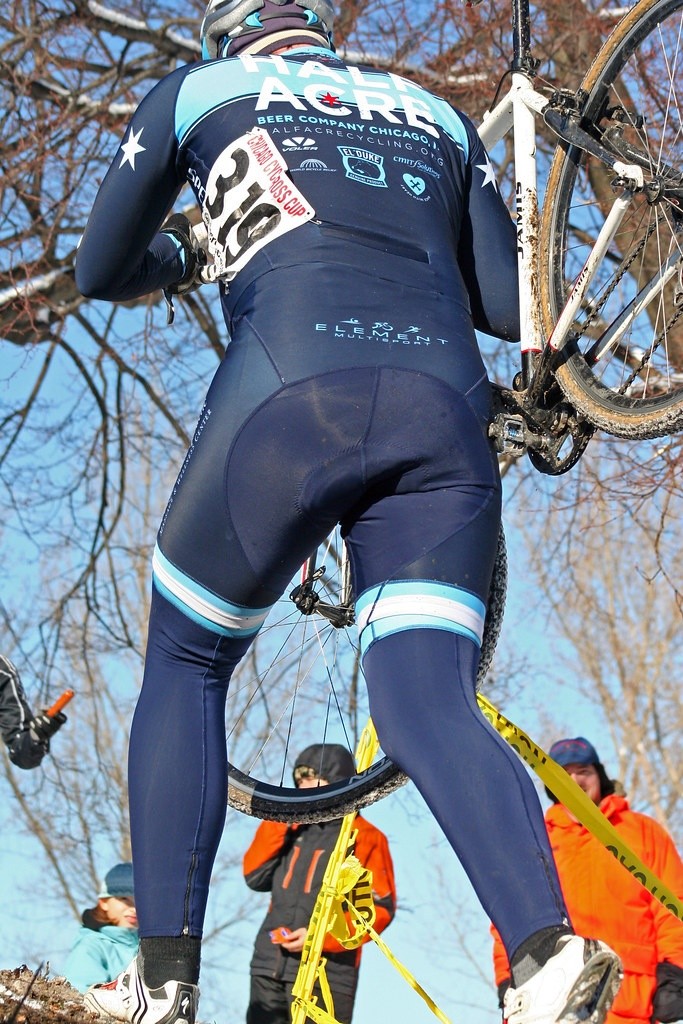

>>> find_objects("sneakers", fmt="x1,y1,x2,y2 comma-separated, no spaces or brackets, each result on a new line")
81,952,200,1024
503,935,624,1024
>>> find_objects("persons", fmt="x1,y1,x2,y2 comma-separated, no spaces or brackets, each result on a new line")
0,654,68,770
489,737,682,1022
74,0,623,1024
63,863,139,994
244,744,396,1024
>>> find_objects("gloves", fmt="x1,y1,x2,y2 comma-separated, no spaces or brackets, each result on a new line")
30,708,67,740
162,211,212,289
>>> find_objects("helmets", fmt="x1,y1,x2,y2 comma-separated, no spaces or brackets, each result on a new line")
199,1,336,57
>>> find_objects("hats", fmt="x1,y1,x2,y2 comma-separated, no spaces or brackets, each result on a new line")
293,743,357,787
544,736,616,802
97,862,135,898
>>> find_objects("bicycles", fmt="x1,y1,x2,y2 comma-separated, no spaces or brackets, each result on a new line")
166,2,682,822
